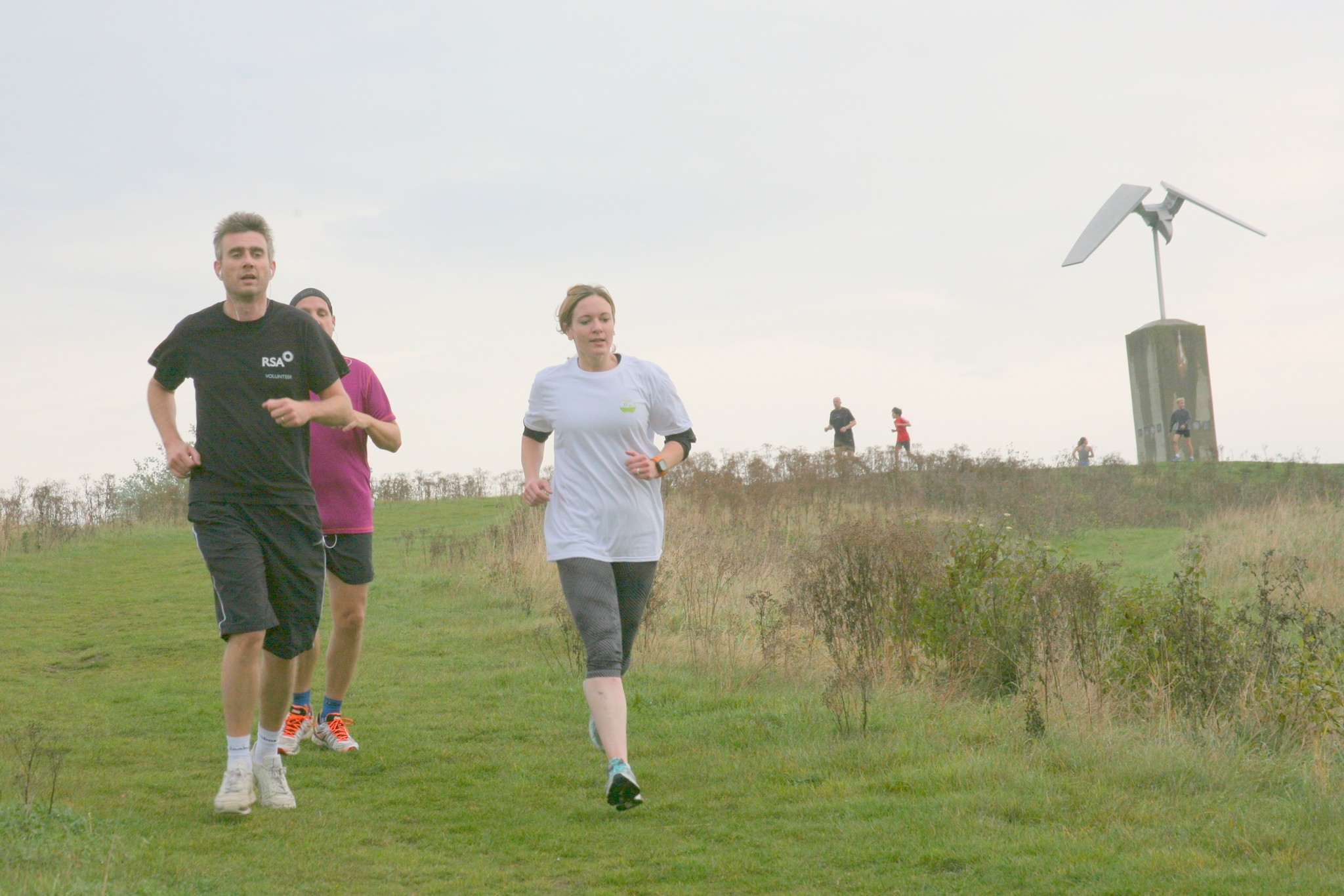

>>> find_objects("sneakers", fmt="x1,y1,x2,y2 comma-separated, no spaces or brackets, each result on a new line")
586,710,604,753
606,757,644,812
250,740,296,812
274,702,315,756
213,759,256,816
310,710,359,754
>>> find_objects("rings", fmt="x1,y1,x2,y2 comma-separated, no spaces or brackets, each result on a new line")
636,467,640,475
281,416,286,423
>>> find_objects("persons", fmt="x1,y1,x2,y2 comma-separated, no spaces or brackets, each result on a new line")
519,285,696,811
891,407,918,470
1168,398,1195,462
1072,437,1094,467
825,397,868,475
274,288,401,759
146,212,352,818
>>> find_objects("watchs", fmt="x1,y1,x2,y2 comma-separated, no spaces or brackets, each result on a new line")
650,456,669,478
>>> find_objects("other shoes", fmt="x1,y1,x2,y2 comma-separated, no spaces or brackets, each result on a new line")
1188,457,1195,462
1171,455,1181,462
